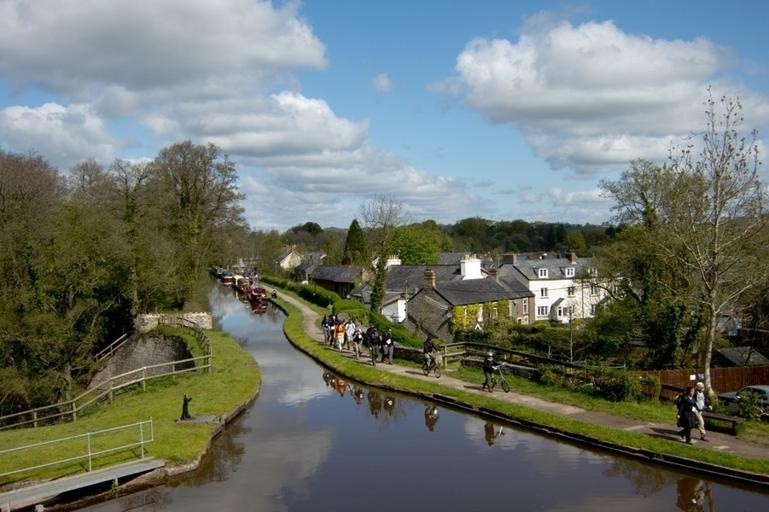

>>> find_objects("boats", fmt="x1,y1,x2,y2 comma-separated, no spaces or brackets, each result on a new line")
248,300,269,316
235,292,249,304
212,268,268,300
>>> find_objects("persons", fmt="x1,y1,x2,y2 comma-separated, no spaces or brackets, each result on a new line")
323,372,395,418
677,476,706,512
692,382,713,442
425,405,439,432
321,315,394,366
676,386,702,444
270,289,277,298
485,422,504,447
482,351,501,392
423,334,440,374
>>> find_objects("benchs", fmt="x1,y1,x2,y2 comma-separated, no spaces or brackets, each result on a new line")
701,410,746,435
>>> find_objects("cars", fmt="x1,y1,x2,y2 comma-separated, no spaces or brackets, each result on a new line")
716,384,769,414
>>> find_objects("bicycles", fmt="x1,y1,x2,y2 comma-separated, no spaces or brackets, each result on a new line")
425,403,440,420
481,361,511,393
488,421,505,439
421,349,443,379
